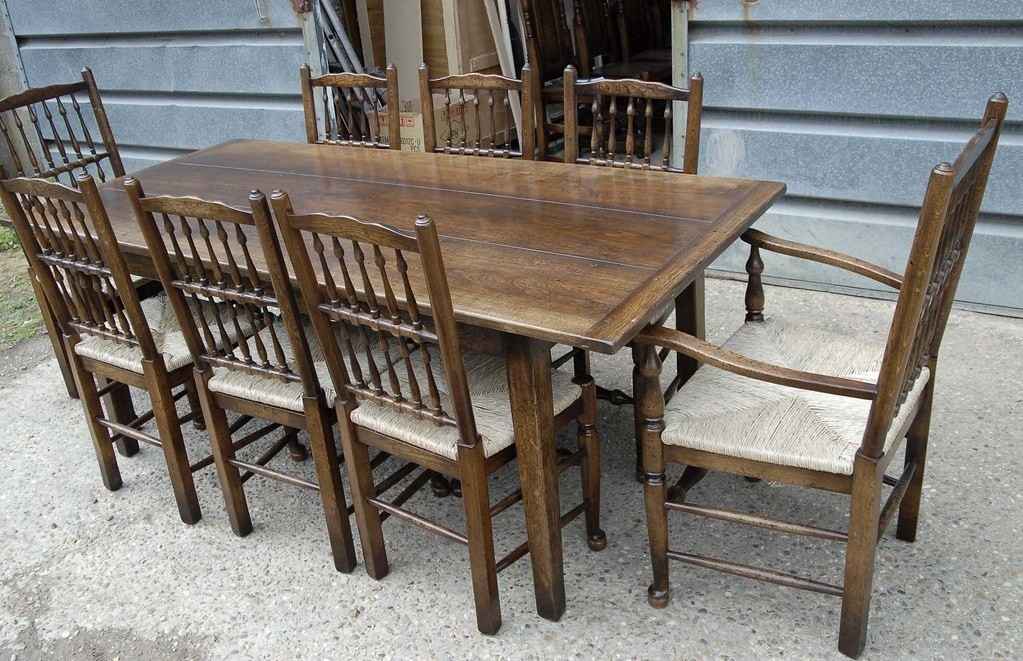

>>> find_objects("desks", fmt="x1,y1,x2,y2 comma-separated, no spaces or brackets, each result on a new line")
0,137,789,625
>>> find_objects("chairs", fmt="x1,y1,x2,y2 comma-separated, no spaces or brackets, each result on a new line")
301,63,400,150
418,62,534,163
0,164,309,526
126,174,450,576
270,189,607,636
549,65,706,484
631,91,1009,661
0,66,177,405
